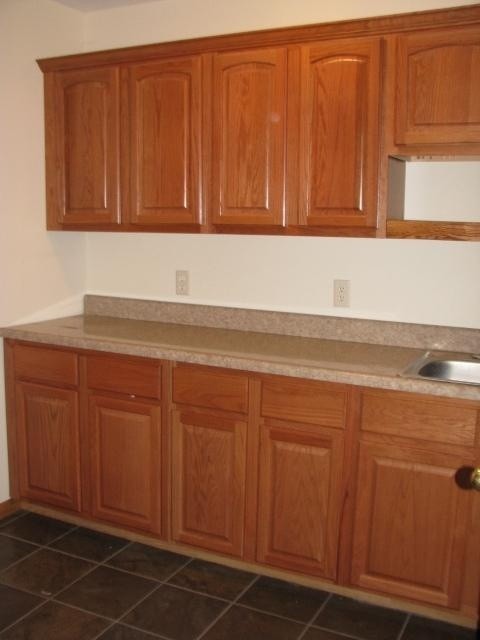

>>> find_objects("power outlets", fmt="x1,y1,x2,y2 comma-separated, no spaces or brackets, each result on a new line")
175,270,188,295
334,279,348,306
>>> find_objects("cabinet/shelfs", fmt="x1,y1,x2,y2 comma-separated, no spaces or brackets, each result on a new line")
4,339,170,550
386,3,480,162
35,33,209,234
351,383,480,630
214,15,405,239
170,363,352,600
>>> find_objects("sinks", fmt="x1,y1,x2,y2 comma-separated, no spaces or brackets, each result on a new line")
398,349,480,386
